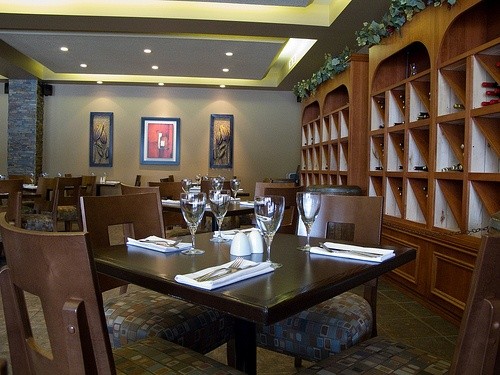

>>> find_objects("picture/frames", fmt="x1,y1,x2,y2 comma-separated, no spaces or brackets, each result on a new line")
210,114,234,169
89,112,113,167
140,116,180,166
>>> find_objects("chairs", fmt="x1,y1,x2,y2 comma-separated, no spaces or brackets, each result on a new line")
0,174,500,375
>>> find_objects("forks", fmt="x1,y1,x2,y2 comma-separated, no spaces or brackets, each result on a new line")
194,257,244,282
145,237,183,247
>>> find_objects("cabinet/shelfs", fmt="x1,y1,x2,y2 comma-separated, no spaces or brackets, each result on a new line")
366,0,500,328
299,54,368,197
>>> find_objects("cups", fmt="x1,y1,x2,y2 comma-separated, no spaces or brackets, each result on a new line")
229,233,251,256
211,178,224,193
248,230,264,254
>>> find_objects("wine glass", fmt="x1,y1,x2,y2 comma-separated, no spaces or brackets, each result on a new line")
229,179,241,202
253,195,286,268
296,192,322,252
179,191,207,254
209,193,227,243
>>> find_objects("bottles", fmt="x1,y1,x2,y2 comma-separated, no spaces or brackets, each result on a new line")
374,62,500,196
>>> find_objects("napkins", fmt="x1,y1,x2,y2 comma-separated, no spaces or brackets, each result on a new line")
214,227,263,241
219,194,241,200
309,241,395,263
239,201,255,206
162,199,180,204
174,259,275,290
125,235,192,253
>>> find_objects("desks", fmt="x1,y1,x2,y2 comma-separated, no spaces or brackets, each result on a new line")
96,181,121,196
160,197,291,232
0,193,42,199
23,184,53,192
90,229,416,375
214,189,249,198
